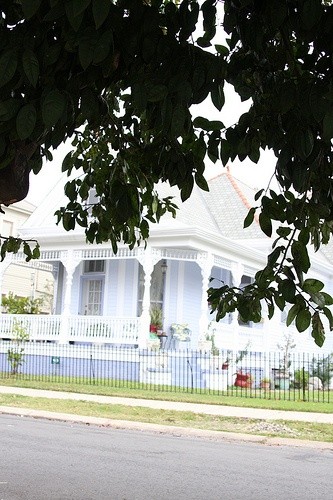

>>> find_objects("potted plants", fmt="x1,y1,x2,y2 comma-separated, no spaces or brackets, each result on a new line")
261,379,270,388
150,308,163,333
277,360,296,390
